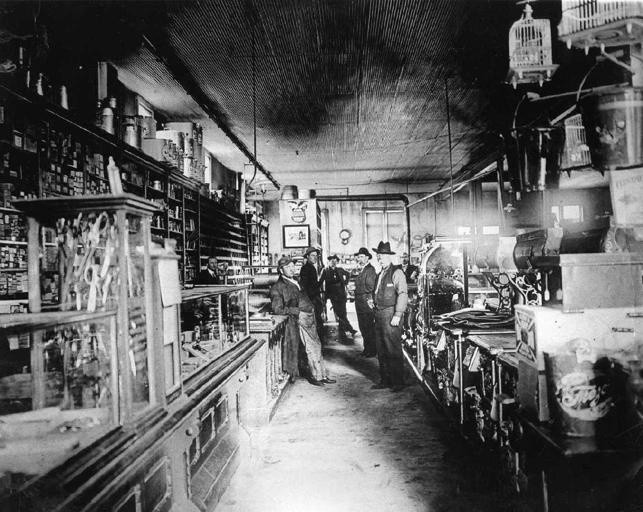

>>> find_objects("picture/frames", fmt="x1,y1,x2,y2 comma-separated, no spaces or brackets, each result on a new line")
283,224,310,248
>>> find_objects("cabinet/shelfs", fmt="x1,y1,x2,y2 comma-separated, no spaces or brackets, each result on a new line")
0,81,289,512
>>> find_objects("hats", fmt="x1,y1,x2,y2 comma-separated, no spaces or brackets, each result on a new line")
301,247,320,258
276,257,297,272
371,241,395,254
327,254,339,261
353,248,371,259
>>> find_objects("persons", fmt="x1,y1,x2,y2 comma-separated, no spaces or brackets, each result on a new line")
268,256,337,386
317,254,357,341
369,240,410,393
196,255,228,323
352,246,379,359
297,246,329,348
396,250,417,283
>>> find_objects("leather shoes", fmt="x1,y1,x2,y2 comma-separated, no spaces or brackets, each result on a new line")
359,351,367,355
389,385,402,392
311,379,324,386
322,377,335,384
369,382,387,389
